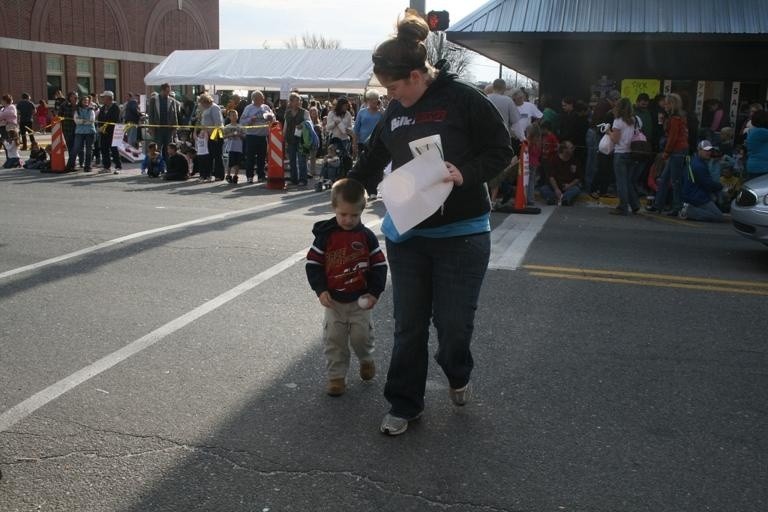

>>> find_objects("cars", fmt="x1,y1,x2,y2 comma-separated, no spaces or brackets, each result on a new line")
730,175,768,244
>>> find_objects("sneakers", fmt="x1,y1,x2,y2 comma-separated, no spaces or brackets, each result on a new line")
68,163,120,175
328,379,345,395
608,207,625,215
198,176,265,184
358,366,376,380
449,379,471,406
678,200,689,221
379,410,409,436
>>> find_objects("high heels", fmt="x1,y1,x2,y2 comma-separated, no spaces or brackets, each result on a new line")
644,205,663,214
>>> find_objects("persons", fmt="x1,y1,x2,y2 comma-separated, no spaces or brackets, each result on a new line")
347,15,512,437
306,178,388,395
1,83,390,192
484,79,767,222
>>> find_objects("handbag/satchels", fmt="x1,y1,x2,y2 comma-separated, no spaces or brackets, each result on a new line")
630,134,651,153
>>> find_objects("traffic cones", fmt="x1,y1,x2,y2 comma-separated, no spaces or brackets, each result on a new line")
516,142,529,209
49,117,66,171
265,121,286,189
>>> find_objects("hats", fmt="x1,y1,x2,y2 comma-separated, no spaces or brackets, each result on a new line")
99,90,115,98
605,90,621,100
696,139,717,152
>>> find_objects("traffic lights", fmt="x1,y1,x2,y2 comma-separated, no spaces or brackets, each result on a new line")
428,10,449,31
